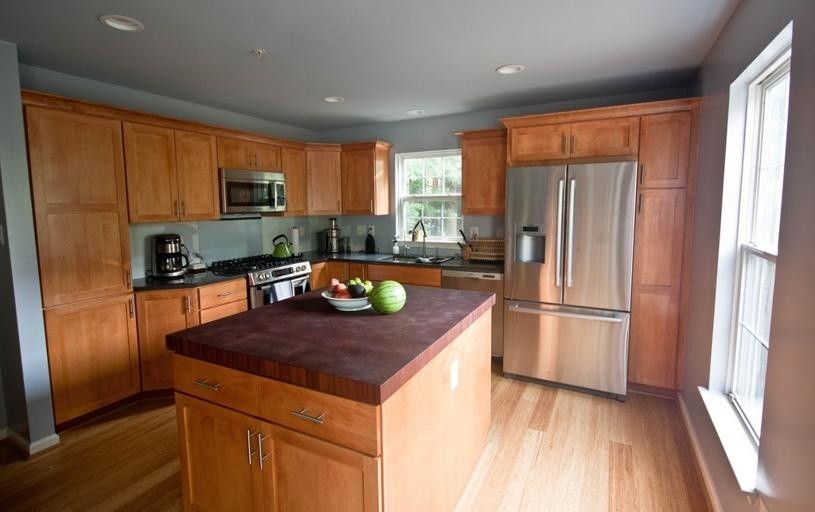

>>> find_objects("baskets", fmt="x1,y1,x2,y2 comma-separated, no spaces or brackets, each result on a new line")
469,239,505,261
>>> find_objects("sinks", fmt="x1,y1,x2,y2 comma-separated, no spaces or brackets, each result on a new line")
378,254,418,264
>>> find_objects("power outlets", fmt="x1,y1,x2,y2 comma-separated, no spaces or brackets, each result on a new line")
368,225,375,237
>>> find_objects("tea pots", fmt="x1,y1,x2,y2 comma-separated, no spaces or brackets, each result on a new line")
271,234,294,260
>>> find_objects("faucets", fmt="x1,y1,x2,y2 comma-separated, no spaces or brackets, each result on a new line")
400,229,410,257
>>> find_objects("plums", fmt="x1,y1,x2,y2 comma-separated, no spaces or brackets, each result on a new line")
352,285,365,298
348,285,354,293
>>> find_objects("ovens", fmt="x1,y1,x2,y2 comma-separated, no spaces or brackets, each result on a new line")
250,274,311,309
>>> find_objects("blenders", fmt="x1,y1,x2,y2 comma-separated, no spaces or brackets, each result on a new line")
324,218,352,255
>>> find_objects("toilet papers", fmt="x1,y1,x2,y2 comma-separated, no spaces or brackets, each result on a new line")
290,229,300,256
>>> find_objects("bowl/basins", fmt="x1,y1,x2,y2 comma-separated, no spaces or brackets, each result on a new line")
321,289,373,312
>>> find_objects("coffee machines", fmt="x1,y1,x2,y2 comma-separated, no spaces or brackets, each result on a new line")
149,232,189,279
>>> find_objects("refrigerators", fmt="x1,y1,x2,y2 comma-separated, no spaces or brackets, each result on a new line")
504,161,639,391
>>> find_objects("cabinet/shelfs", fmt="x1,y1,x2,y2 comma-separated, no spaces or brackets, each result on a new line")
350,263,368,279
18,91,141,428
215,126,281,169
624,94,700,399
135,290,198,392
338,140,394,218
499,104,641,164
328,262,348,286
311,262,327,291
264,139,307,218
170,306,492,512
453,126,508,217
200,277,249,325
369,265,441,287
120,106,221,223
306,142,341,217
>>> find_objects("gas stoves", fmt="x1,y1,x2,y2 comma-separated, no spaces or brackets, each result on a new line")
210,253,314,286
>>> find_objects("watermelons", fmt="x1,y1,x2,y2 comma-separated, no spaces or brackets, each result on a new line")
369,280,407,314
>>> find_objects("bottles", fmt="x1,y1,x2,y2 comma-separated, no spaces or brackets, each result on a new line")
366,234,375,254
392,241,400,255
292,226,300,256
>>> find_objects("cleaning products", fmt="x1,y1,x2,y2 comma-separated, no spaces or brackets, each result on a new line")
392,235,399,254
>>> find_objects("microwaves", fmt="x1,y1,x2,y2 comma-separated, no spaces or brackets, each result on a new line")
219,169,289,215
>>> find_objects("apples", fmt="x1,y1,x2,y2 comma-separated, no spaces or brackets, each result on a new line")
327,278,339,292
335,289,351,298
335,283,347,292
